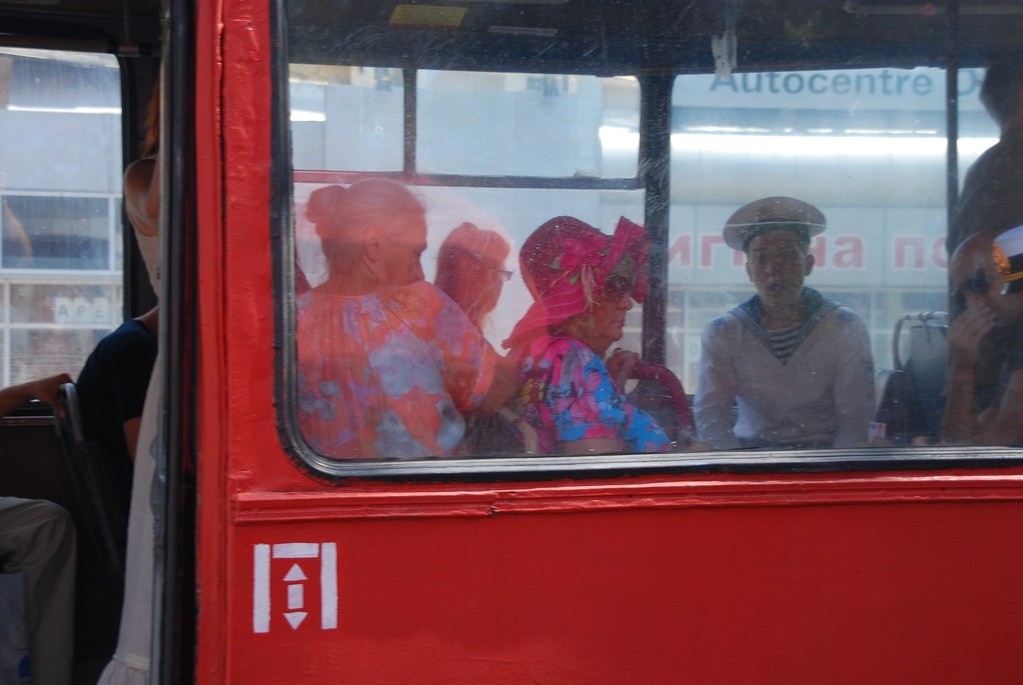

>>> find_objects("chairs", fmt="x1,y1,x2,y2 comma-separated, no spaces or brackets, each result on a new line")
54,311,949,578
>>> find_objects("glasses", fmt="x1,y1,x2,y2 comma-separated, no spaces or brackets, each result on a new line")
490,266,513,282
948,267,999,308
599,274,635,303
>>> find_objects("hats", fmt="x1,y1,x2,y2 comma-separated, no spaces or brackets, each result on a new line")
992,225,1023,295
723,196,827,254
502,216,651,349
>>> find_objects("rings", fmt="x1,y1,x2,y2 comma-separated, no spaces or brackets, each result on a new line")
613,347,623,354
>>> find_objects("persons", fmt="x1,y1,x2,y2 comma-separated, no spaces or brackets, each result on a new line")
296,180,551,460
433,222,518,458
943,225,1023,446
694,196,875,452
0,372,78,685
506,216,673,457
945,46,1023,254
67,305,158,569
